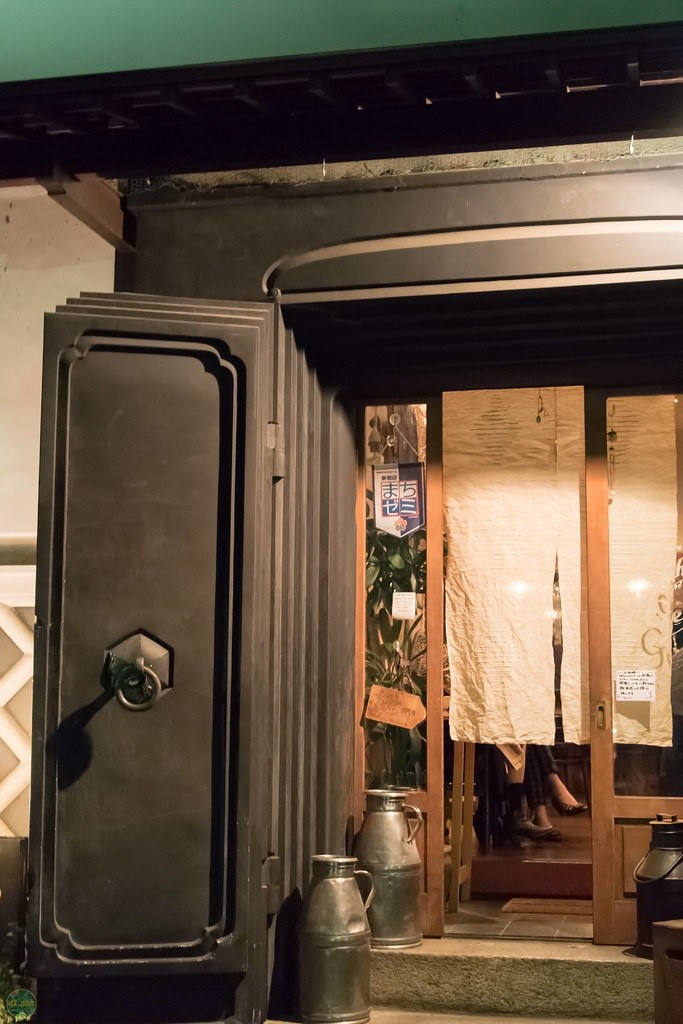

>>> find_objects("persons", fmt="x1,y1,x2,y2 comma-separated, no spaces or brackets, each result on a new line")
495,743,584,842
660,646,683,797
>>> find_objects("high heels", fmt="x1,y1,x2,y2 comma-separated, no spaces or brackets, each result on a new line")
530,816,563,843
551,795,589,817
502,812,556,850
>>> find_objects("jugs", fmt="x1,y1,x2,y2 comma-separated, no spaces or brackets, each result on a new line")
632,812,683,954
353,789,423,949
293,853,377,1024
